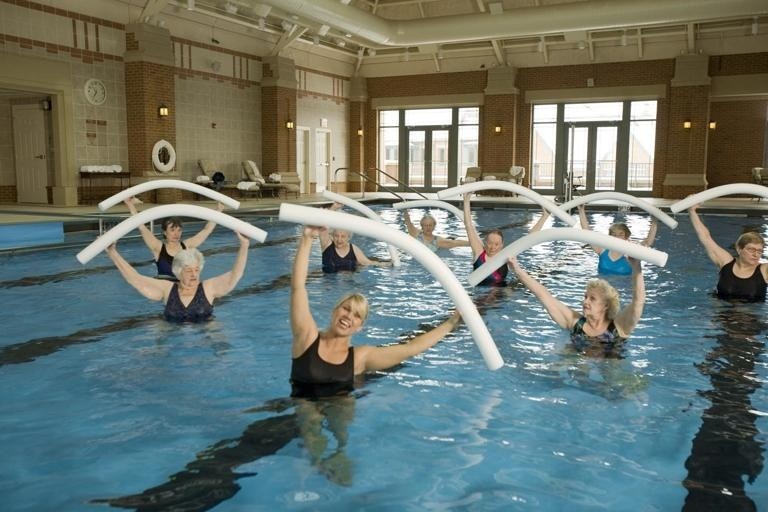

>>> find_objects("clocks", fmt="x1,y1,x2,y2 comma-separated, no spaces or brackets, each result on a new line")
84,79,107,106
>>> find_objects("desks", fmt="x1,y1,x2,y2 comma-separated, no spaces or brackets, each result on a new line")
79,172,130,205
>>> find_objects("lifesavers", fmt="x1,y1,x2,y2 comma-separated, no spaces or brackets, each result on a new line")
152,140,176,173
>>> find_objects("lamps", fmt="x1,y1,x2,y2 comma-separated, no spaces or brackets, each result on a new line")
684,119,692,129
358,128,363,136
159,104,168,117
285,119,294,129
495,123,502,133
709,121,717,129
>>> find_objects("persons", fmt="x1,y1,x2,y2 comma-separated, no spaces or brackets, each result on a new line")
289,225,464,402
96,229,251,321
318,200,393,273
576,202,658,275
122,194,227,281
461,190,551,286
683,192,768,303
508,255,648,358
403,209,470,254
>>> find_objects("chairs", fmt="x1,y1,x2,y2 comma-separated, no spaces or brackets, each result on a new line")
195,158,301,201
751,165,768,203
460,165,525,198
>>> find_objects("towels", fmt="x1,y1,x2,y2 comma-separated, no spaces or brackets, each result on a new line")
80,165,123,174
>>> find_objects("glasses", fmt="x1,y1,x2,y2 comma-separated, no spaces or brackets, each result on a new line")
747,247,763,254
